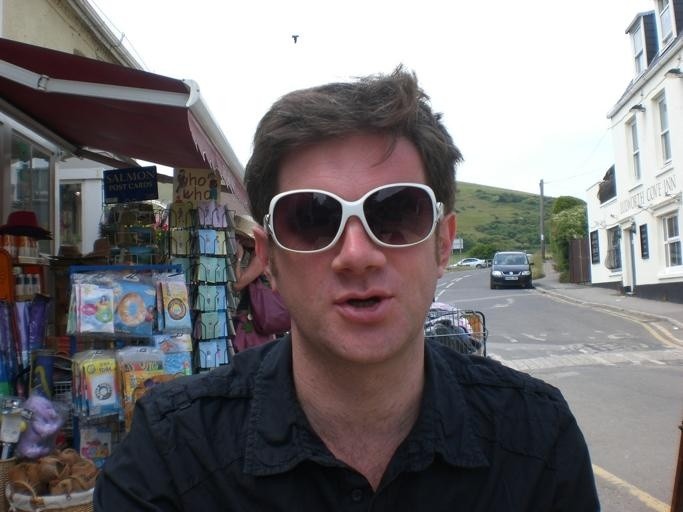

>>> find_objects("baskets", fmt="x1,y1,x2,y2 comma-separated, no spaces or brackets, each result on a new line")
0,456,17,512
5,480,95,512
423,307,489,358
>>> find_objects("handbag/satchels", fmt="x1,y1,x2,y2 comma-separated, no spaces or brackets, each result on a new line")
248,276,291,336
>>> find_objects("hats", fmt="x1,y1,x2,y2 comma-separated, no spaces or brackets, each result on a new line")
235,215,260,243
48,243,86,262
0,211,55,241
83,239,114,259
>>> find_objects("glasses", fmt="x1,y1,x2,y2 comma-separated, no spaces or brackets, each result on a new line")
261,183,447,255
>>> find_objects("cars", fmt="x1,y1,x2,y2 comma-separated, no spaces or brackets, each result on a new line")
450,258,487,269
488,251,534,289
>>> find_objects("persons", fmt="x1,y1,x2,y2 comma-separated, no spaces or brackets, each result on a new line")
91,62,603,510
